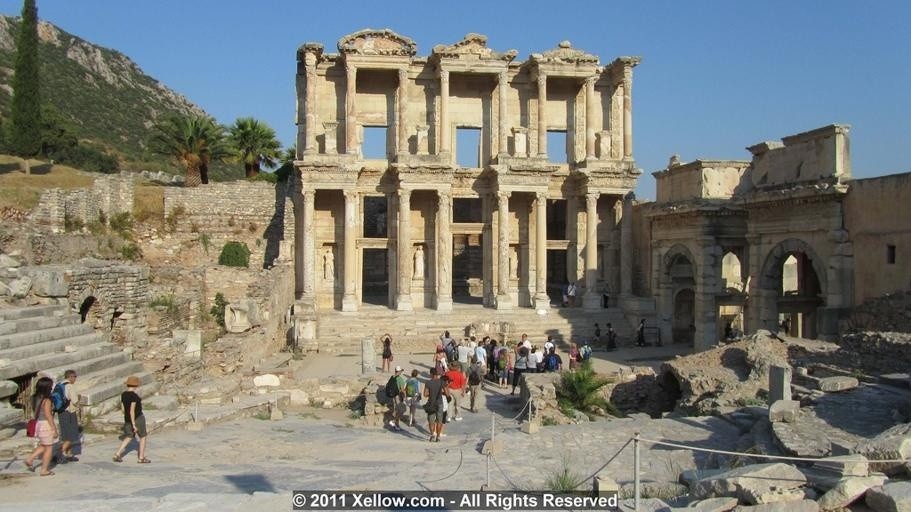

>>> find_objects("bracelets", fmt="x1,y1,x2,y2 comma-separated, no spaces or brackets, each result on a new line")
131,422,136,425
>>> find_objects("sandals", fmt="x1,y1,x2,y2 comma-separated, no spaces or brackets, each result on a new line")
111,454,122,462
136,457,150,463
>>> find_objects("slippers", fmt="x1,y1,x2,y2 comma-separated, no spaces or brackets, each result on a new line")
24,461,35,472
39,472,54,476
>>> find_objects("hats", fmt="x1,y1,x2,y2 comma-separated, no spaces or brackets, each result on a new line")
448,361,460,370
123,376,141,386
394,366,403,372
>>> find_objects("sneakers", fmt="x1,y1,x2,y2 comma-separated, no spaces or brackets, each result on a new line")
429,435,441,442
388,421,401,431
446,417,462,421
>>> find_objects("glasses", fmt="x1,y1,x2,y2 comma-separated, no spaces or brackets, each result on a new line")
71,376,75,378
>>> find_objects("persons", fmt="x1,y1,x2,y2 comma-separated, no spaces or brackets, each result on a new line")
568,342,579,374
580,339,593,364
424,323,563,441
389,367,405,431
58,370,82,460
114,377,152,464
25,377,60,475
638,318,648,348
380,333,393,372
784,319,790,334
602,281,610,308
606,323,617,349
592,323,601,343
725,323,733,341
403,369,419,426
568,281,576,308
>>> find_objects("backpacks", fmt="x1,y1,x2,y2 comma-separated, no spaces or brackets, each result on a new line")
385,375,399,398
405,384,415,397
547,355,557,369
468,367,480,385
498,359,506,370
583,346,591,359
50,382,70,416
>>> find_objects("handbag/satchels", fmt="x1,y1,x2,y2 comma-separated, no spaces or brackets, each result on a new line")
423,402,438,414
26,420,35,437
